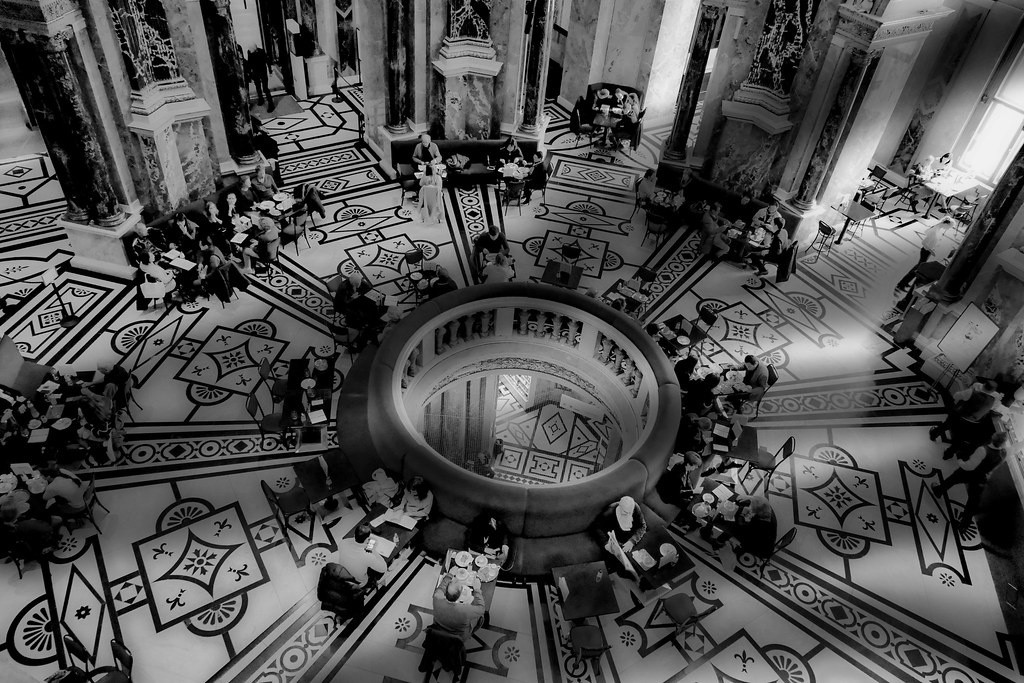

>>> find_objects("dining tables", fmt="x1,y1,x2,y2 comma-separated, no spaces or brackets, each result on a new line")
710,420,759,473
601,278,654,312
619,526,695,596
12,469,52,519
434,549,501,612
280,357,335,426
34,371,97,406
17,406,78,444
657,314,707,350
342,504,419,564
293,449,361,504
551,560,621,621
701,363,750,395
476,253,516,278
352,288,386,312
406,269,435,296
687,477,751,531
541,261,584,290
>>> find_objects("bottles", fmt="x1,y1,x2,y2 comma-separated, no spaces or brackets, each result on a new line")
393,533,399,548
596,569,603,583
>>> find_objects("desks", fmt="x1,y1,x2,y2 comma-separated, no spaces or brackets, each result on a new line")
723,225,766,269
922,176,978,219
397,160,497,183
652,190,682,213
593,113,624,147
176,193,303,301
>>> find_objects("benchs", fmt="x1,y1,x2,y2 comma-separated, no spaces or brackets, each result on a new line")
0,335,53,399
119,186,229,268
391,140,537,171
656,162,803,241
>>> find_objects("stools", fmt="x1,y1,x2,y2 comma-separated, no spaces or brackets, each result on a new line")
401,180,419,206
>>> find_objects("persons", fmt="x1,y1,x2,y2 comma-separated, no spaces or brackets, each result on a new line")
1,36,1011,648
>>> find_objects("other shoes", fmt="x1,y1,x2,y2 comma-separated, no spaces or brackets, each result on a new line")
953,519,966,534
895,286,908,296
929,426,937,441
943,452,954,460
755,270,768,276
932,483,943,498
905,282,912,288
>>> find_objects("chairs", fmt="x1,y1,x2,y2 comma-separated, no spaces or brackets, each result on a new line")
0,335,145,579
805,221,836,259
847,199,876,241
145,183,317,315
894,174,923,212
950,194,989,227
569,82,647,156
831,199,876,244
630,177,798,283
502,161,555,216
244,231,796,675
419,185,441,223
44,635,133,683
869,164,897,211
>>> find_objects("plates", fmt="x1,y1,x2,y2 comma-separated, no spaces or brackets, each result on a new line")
410,273,422,280
702,493,715,504
455,551,472,566
660,543,677,557
456,568,469,580
477,564,499,582
301,379,316,389
475,556,488,567
726,371,744,383
677,336,690,345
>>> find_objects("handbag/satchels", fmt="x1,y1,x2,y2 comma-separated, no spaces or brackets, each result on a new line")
140,273,165,298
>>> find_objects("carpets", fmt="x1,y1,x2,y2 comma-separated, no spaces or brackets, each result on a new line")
248,96,305,120
